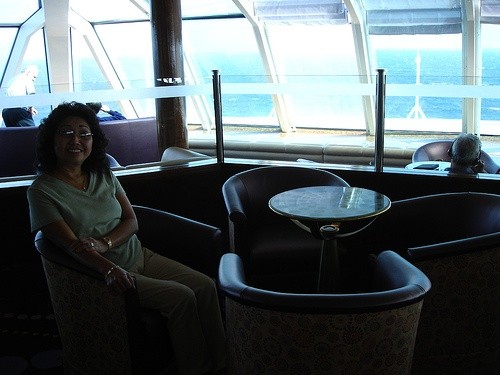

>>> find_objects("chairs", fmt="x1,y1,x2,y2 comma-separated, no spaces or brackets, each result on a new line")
34,140,500,375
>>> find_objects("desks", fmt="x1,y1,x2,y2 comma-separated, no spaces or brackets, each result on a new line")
405,161,452,171
268,185,392,294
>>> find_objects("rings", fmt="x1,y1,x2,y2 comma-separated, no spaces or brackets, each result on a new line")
90,242,94,246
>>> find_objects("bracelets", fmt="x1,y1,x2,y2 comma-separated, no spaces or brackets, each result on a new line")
105,264,120,279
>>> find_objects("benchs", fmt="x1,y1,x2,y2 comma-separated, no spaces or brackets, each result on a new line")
0,117,161,177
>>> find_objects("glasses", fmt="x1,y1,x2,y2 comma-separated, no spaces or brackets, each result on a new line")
54,129,94,140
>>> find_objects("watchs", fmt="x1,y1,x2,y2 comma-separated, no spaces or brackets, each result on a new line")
100,237,113,251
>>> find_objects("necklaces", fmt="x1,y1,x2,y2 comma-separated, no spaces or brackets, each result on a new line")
80,185,87,190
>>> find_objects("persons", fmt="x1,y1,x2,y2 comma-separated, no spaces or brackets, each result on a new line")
86,102,126,122
444,133,487,173
27,101,226,375
2,65,38,127
